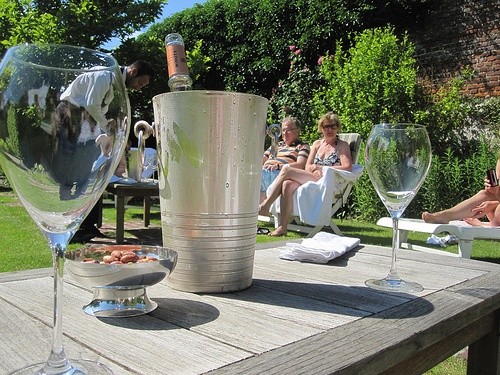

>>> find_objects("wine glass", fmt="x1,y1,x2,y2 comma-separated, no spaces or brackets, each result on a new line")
363,122,432,292
1,44,131,375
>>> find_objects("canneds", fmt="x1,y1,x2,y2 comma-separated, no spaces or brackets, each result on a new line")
486,170,497,186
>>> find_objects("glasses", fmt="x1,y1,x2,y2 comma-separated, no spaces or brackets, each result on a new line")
321,124,339,130
281,128,297,134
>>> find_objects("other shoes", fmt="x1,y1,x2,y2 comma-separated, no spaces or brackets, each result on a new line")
68,229,94,244
86,226,107,238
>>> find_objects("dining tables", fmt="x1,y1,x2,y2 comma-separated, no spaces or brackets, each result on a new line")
0,239,500,375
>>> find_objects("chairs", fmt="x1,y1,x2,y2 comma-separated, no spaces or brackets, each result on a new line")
257,133,364,239
377,217,500,258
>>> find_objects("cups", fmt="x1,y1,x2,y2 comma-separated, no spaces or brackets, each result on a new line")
486,169,498,187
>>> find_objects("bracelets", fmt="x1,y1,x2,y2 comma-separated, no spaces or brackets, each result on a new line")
277,164,279,170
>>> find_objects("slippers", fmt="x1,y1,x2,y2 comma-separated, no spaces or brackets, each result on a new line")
256,227,270,233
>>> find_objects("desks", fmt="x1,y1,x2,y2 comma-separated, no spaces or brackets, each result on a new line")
97,182,159,245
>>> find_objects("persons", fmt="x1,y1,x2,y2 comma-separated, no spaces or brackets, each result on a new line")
258,114,352,236
95,118,125,177
56,66,114,200
56,60,155,244
422,159,500,226
259,117,310,204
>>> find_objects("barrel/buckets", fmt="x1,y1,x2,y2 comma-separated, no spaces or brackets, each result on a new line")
151,91,268,293
128,148,157,180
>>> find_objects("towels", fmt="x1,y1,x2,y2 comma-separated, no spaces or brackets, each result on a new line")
426,234,455,247
267,164,364,226
117,177,137,184
131,148,155,178
279,231,360,263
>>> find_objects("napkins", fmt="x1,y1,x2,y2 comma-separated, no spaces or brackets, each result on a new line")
279,232,361,264
